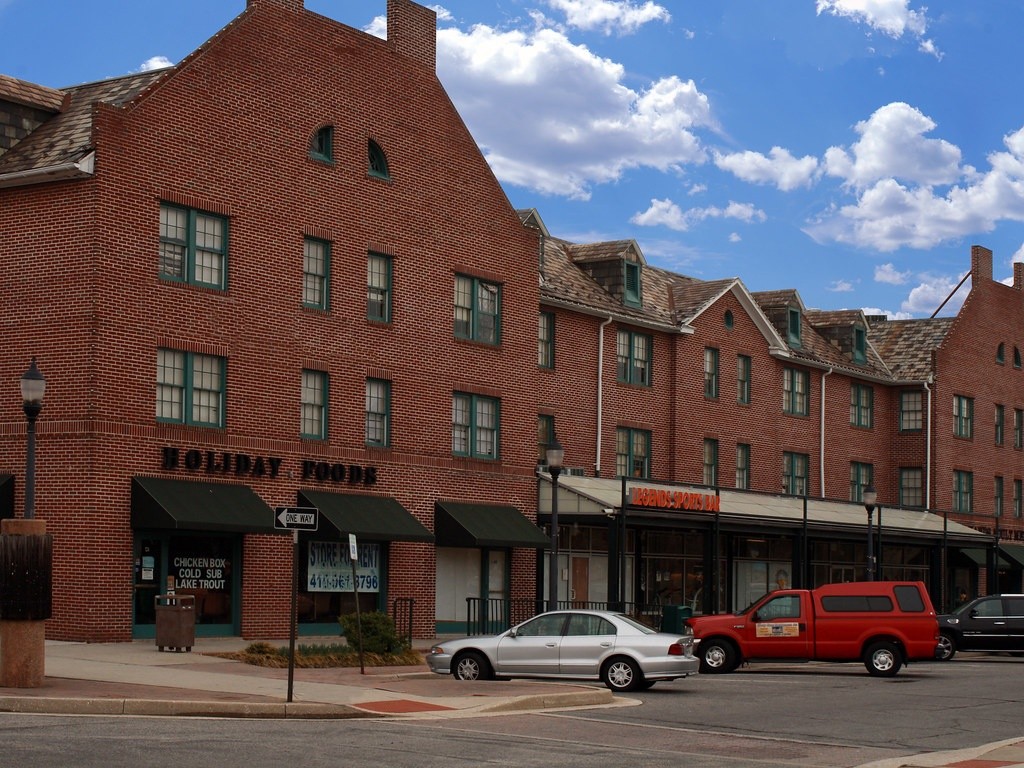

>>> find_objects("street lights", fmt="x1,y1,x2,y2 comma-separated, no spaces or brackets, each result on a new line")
862,480,879,583
545,432,565,610
18,355,47,520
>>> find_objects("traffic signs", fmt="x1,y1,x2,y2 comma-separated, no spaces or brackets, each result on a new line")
275,505,318,532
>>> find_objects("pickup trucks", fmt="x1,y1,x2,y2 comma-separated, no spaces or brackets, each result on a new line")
683,579,940,680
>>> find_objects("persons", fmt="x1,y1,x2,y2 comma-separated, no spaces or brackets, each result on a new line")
774,569,789,590
959,588,970,603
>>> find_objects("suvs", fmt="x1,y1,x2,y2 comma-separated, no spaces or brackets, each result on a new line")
936,593,1024,663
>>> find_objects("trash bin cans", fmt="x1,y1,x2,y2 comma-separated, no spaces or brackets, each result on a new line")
155,594,197,653
660,604,693,634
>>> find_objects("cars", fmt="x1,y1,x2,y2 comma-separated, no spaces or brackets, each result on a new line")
425,608,702,693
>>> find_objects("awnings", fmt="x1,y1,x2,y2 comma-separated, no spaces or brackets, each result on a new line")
130,474,293,538
295,488,436,544
431,498,557,551
